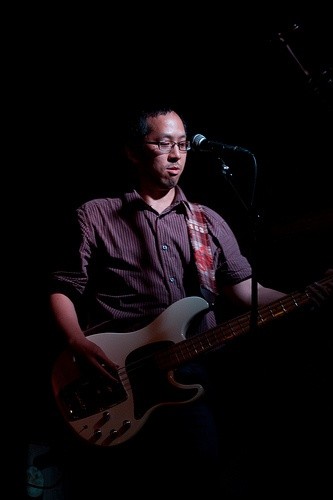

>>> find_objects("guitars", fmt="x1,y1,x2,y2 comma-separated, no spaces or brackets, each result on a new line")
51,272,333,446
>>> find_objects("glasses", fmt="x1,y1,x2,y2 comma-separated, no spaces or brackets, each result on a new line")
140,136,191,152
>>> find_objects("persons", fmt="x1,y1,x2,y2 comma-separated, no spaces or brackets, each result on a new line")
47,101,333,500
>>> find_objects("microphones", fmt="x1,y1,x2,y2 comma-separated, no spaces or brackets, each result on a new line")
191,134,252,156
